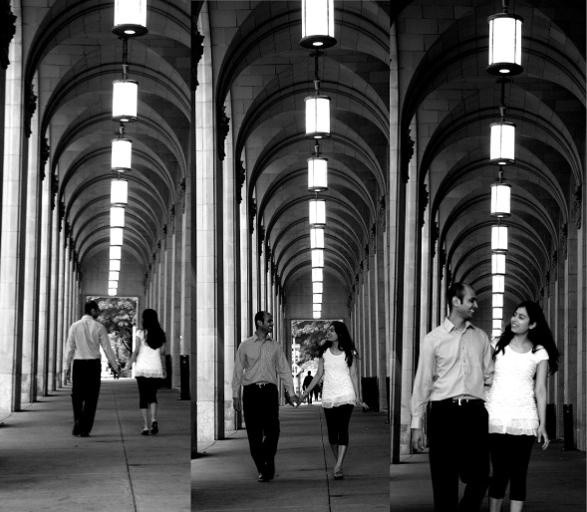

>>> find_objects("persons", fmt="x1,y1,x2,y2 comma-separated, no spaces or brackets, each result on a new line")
119,307,166,435
301,370,314,404
230,310,299,483
297,319,370,480
483,299,560,511
313,383,320,401
408,281,495,512
61,300,121,437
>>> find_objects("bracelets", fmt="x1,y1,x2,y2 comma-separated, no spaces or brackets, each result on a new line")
302,390,309,398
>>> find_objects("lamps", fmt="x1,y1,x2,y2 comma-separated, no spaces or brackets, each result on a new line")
110,0,148,38
297,0,337,50
484,0,524,77
107,64,138,295
485,108,516,350
304,80,330,322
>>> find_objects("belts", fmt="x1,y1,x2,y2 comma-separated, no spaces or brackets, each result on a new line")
256,382,267,388
452,397,482,407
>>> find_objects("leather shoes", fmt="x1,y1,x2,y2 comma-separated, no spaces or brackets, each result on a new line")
255,458,275,482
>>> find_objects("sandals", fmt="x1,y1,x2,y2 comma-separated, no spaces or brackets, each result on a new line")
333,468,342,478
150,420,159,434
141,427,149,434
